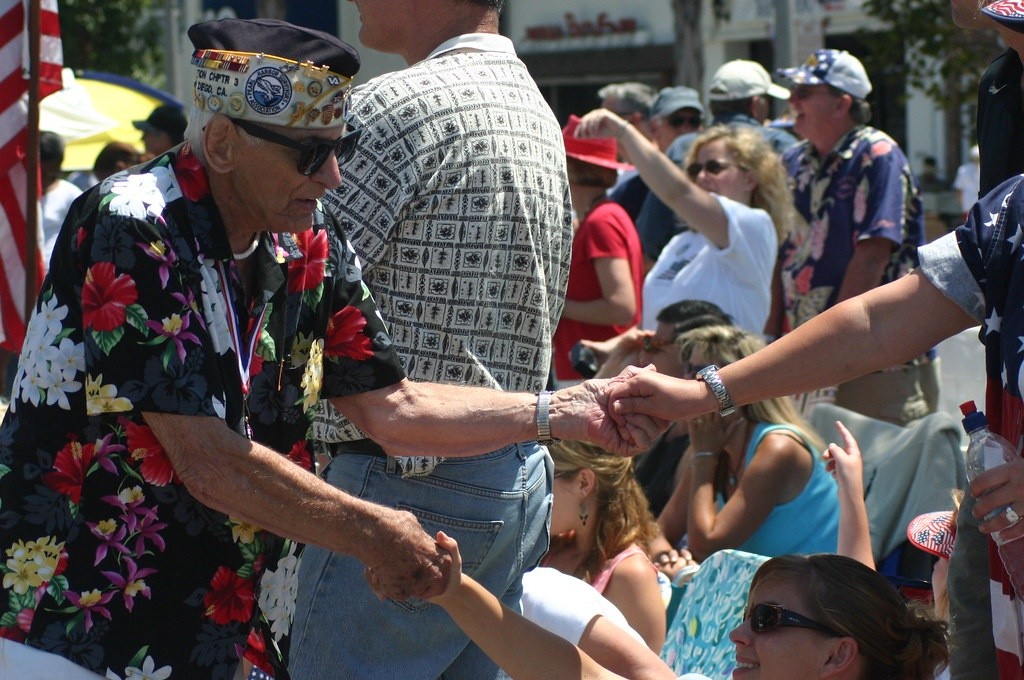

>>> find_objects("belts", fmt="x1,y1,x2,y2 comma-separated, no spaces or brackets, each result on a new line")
308,438,386,458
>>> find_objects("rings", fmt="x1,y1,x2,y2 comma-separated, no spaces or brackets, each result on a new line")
1005,506,1019,523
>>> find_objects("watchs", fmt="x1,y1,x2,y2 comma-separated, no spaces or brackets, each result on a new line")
695,364,735,418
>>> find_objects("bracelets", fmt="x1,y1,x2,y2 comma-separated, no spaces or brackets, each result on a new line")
533,390,561,446
692,452,719,457
614,120,629,139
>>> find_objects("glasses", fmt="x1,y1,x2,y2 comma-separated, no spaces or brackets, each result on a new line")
670,116,700,129
687,159,725,176
744,603,845,638
237,117,363,176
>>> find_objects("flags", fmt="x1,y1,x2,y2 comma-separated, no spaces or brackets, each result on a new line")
0,0,66,359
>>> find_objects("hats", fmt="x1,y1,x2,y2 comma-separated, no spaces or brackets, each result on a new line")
779,48,872,101
710,57,791,102
907,510,958,558
132,105,187,133
185,18,361,129
649,85,705,117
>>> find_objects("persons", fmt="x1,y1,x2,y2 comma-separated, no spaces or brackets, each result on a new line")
0,0,1024,680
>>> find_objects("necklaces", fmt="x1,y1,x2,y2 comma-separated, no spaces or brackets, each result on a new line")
726,423,751,486
232,231,260,260
580,193,605,222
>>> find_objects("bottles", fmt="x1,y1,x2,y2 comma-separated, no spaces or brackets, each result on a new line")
960,399,1024,606
